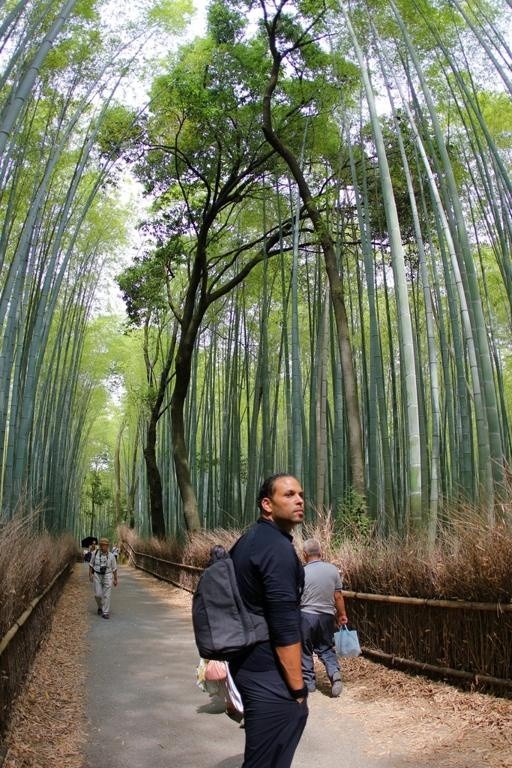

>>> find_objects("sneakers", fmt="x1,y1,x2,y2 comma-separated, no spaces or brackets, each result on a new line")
330,671,342,697
98,608,109,619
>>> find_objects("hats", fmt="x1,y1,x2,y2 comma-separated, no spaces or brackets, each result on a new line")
99,538,109,545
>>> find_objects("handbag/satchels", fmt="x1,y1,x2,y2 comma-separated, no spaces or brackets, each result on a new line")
334,625,362,659
206,660,227,681
85,552,92,561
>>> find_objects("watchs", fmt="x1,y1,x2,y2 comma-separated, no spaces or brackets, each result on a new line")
290,683,308,699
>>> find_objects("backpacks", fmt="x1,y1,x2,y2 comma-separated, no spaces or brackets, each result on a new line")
192,543,269,663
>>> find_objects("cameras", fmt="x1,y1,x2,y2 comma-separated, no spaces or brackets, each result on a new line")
100,566,106,575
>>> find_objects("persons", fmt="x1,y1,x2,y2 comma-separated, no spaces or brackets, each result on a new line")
205,546,245,729
229,471,309,768
82,537,121,620
299,538,347,697
